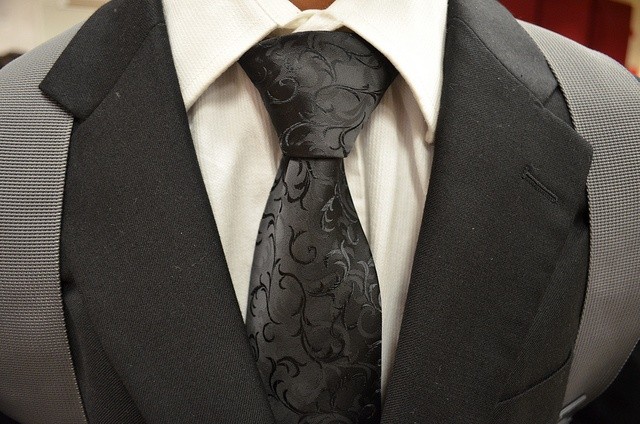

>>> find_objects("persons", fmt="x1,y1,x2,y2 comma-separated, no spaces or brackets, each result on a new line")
0,0,639,424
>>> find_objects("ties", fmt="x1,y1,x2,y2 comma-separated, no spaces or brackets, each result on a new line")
237,26,399,424
